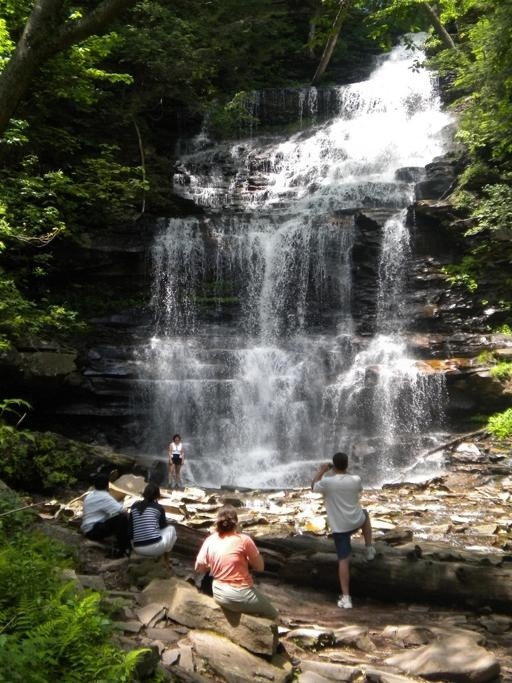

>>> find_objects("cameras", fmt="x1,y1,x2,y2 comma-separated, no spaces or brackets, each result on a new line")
326,462,333,471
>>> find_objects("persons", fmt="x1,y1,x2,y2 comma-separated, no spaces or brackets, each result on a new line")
81,474,130,548
128,483,178,569
194,504,279,628
168,434,185,486
311,452,376,609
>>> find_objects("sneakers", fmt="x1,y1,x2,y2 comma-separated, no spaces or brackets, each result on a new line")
336,596,352,610
366,545,377,561
